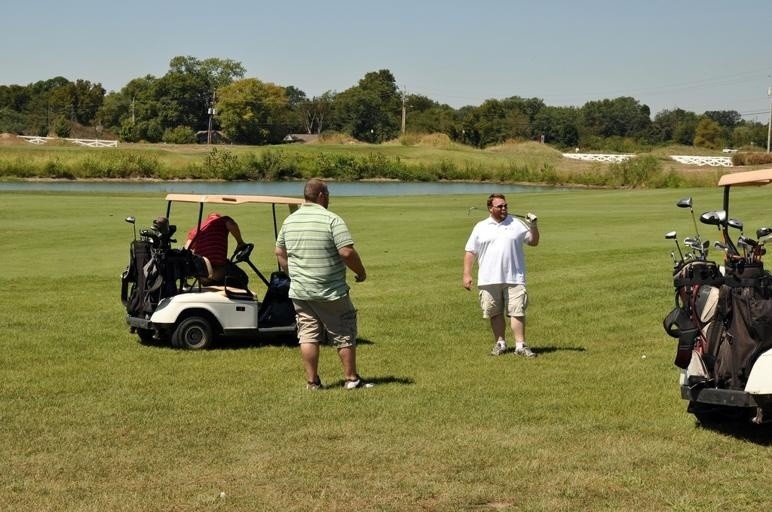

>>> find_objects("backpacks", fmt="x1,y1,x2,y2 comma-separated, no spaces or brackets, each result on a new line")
661,254,772,388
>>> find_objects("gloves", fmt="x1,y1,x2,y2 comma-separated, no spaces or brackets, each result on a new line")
525,212,538,228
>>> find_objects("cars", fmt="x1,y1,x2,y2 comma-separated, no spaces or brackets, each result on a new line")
196,130,231,143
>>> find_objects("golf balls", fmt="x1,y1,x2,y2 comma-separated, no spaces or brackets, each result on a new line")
641,355,646,359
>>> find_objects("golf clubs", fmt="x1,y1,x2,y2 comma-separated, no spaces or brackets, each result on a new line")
665,197,772,267
124,216,177,243
466,207,537,222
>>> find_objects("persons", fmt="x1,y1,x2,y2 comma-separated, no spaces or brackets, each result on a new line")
463,192,540,358
275,179,376,389
183,211,250,288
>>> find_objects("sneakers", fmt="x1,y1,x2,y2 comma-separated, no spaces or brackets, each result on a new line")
306,376,322,391
344,374,375,390
513,344,537,358
491,343,507,356
